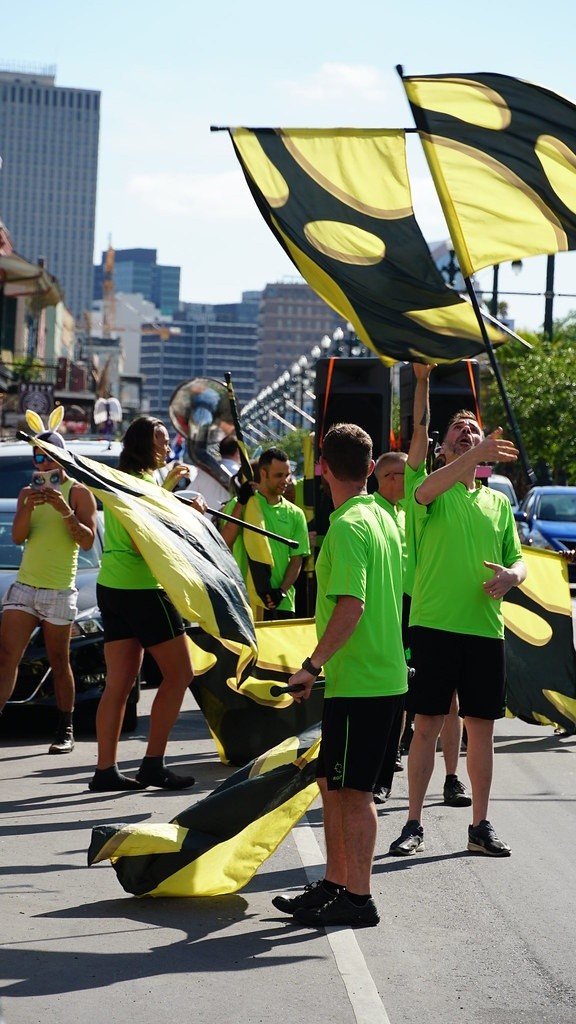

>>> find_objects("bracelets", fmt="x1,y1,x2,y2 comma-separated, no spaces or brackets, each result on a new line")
302,657,321,677
60,510,75,519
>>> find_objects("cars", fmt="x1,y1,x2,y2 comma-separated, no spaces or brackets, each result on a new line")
1,440,168,735
512,485,576,583
489,473,521,519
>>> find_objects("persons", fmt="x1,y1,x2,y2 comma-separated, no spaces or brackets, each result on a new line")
389,408,527,858
539,502,555,520
296,476,318,618
89,416,208,793
272,420,408,926
0,406,97,755
217,458,261,588
372,452,411,773
558,550,576,560
373,364,473,808
221,448,310,621
185,436,243,521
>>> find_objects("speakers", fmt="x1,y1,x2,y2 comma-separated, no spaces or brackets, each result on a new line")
313,357,392,537
400,359,479,474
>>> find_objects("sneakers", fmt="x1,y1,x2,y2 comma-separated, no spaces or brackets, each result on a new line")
467,820,513,857
271,880,336,915
395,747,404,771
444,779,471,807
372,785,392,802
293,889,380,927
390,820,425,855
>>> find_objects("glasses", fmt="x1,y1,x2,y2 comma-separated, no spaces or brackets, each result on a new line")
35,454,55,463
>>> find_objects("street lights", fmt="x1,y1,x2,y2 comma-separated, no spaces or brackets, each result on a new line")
488,248,523,322
237,316,368,449
440,236,467,290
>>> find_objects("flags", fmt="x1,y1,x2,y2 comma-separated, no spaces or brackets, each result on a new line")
229,128,510,368
87,722,320,899
183,618,326,768
402,73,576,279
29,436,258,690
504,544,576,736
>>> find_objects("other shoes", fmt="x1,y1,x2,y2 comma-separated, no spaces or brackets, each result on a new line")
50,725,75,753
136,766,195,788
89,774,145,791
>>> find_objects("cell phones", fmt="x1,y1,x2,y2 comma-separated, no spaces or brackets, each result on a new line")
32,469,61,499
